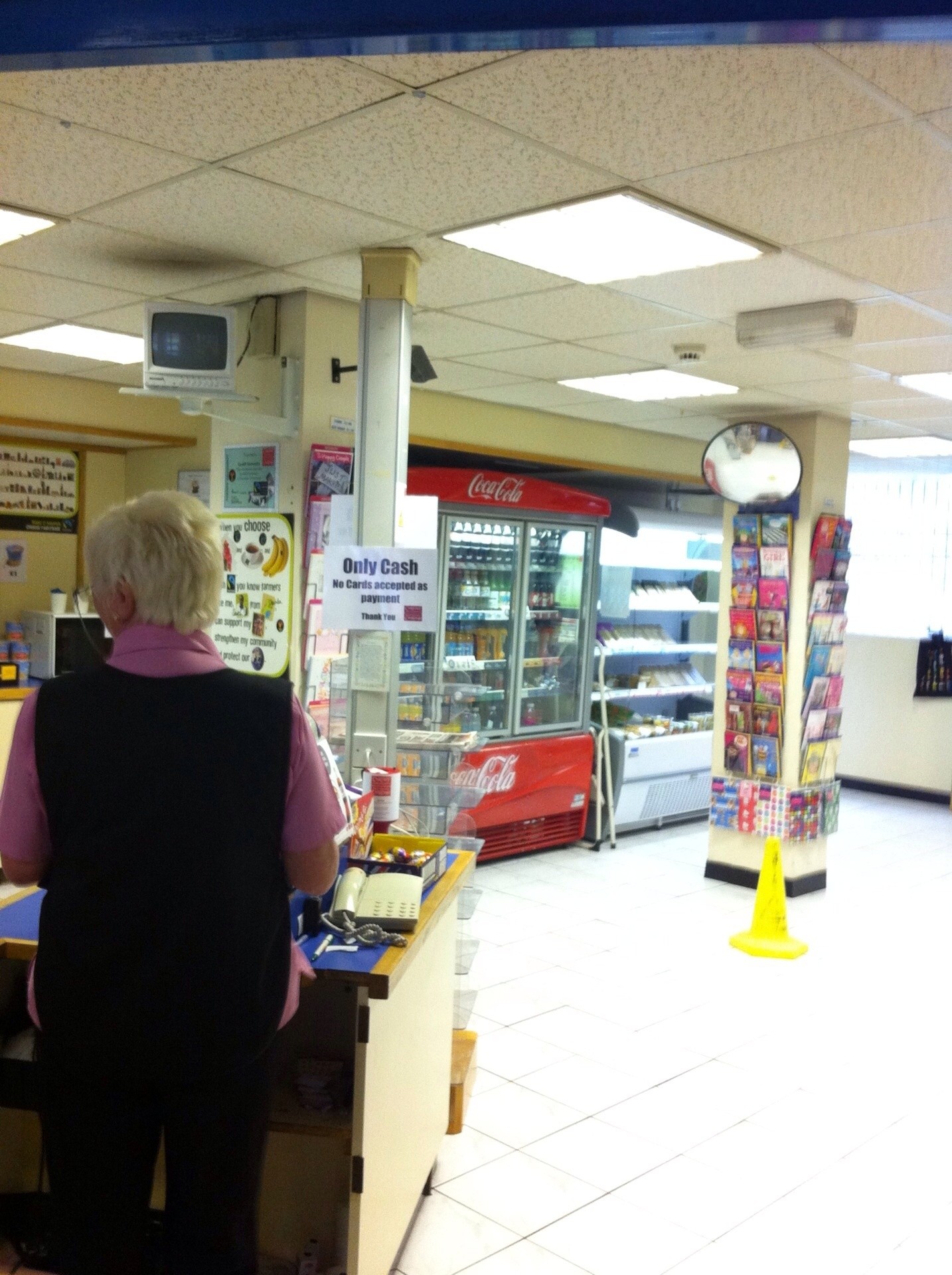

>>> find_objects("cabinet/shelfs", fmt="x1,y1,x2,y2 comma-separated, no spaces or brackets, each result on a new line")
597,554,720,837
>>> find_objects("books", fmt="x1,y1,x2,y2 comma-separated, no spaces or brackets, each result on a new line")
301,444,355,765
723,513,853,784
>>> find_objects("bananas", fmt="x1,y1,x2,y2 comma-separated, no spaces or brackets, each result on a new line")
261,535,289,576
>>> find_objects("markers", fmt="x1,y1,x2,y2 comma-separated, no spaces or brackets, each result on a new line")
312,934,333,961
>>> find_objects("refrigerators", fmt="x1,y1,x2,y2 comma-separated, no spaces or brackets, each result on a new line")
396,469,595,862
585,505,726,841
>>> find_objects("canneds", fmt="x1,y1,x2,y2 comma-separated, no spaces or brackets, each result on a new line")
395,753,421,831
0,621,31,680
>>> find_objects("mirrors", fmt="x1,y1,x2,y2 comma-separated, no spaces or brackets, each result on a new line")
703,421,804,505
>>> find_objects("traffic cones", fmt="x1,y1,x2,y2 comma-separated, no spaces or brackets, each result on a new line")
730,834,810,959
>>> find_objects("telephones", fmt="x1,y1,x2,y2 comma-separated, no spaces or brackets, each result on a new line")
329,866,424,930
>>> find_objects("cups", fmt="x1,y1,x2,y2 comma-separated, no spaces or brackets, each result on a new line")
245,543,260,563
50,592,66,615
0,622,31,689
6,546,24,566
73,592,89,615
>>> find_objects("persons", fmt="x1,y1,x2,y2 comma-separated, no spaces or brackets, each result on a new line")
0,486,347,1275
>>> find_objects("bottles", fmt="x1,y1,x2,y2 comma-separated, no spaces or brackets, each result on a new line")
401,631,427,662
398,695,423,721
447,627,455,656
466,626,474,656
461,706,499,732
523,668,561,691
523,703,536,727
443,671,504,690
498,624,509,660
529,582,554,611
456,626,465,656
526,622,559,658
475,624,487,660
448,568,511,611
488,624,498,660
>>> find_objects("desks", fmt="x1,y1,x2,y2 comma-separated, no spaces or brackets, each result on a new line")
0,850,474,1275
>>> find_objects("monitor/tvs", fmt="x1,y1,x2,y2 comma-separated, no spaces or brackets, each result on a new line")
142,300,238,392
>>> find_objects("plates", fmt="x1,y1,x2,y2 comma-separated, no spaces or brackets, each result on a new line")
241,552,264,569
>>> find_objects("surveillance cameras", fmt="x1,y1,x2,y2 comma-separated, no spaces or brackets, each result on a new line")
410,345,438,385
